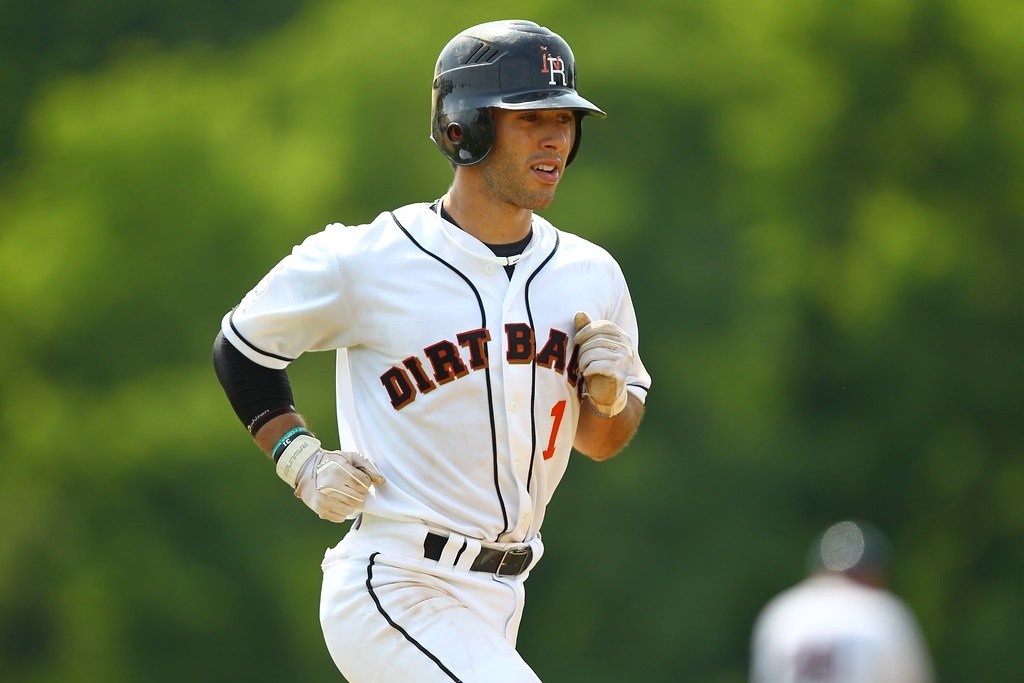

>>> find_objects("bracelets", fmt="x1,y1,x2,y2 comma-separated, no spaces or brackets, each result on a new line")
585,383,628,416
269,425,314,462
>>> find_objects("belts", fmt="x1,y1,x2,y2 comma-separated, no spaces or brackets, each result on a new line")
424,531,533,576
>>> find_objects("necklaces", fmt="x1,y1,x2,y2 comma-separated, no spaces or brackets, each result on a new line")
437,193,540,265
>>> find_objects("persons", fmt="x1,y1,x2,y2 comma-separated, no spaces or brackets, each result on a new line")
750,525,936,683
214,19,651,683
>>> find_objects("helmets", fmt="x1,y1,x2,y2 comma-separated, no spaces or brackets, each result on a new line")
429,17,609,166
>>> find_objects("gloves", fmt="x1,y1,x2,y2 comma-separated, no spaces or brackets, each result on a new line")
567,311,632,420
274,434,385,525
